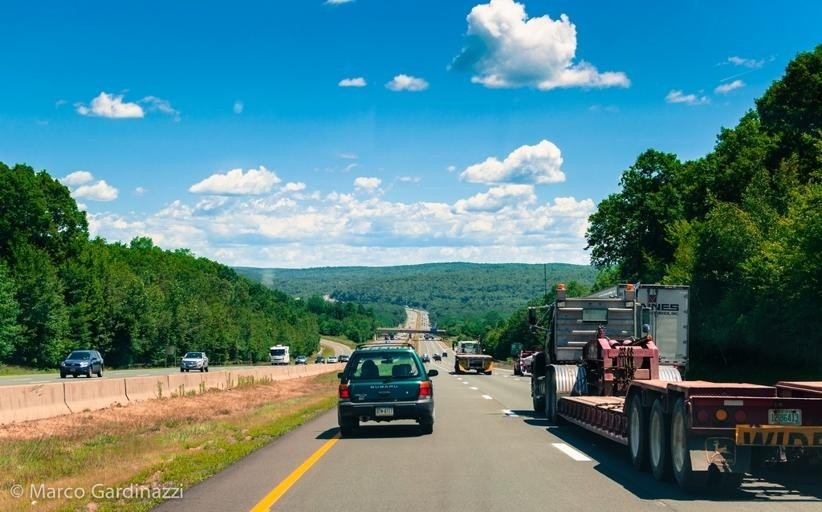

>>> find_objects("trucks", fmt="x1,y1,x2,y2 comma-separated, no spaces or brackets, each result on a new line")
582,282,692,380
525,281,821,499
268,344,291,366
452,338,494,375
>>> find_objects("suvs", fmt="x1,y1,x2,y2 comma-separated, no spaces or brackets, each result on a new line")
179,351,209,372
60,349,105,379
336,342,439,436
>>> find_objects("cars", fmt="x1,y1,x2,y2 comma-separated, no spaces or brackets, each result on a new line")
513,350,544,377
420,351,447,363
385,334,436,342
293,354,349,365
